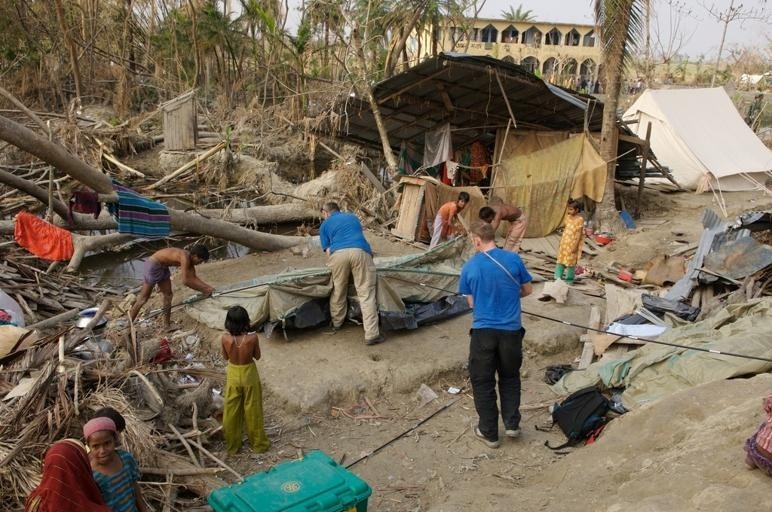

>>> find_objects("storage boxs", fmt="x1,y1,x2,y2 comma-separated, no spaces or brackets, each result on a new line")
206,448,373,512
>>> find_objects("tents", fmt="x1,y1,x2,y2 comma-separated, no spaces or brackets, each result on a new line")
622,83,772,195
739,73,767,83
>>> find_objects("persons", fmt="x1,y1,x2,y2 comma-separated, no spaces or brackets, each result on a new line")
83,408,147,511
318,198,386,346
24,437,111,512
575,77,643,93
457,220,534,449
428,191,470,250
220,305,272,455
478,200,529,254
128,241,217,333
553,196,586,284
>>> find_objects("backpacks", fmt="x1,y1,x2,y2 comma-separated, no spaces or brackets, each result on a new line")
552,387,612,445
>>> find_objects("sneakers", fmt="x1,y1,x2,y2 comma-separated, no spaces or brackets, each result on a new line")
363,333,386,344
472,425,499,450
504,427,521,439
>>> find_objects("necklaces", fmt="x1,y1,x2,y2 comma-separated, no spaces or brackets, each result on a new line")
233,334,246,348
